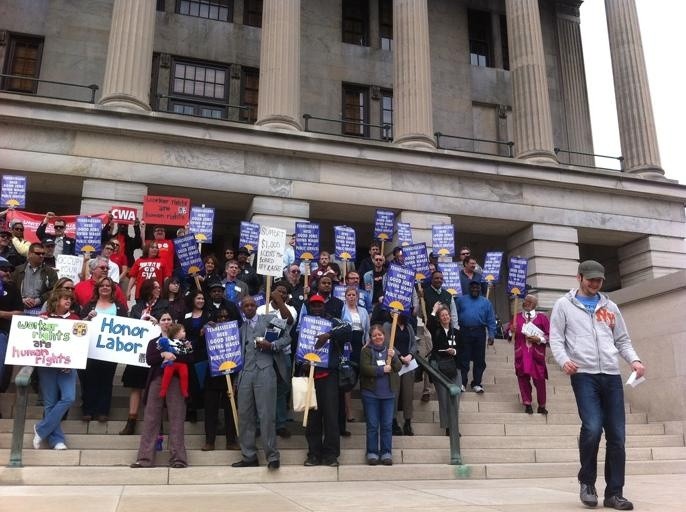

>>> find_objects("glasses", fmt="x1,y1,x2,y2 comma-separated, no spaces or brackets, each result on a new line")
54,225,63,229
32,252,44,255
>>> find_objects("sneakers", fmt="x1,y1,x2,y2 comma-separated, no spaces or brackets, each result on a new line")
370,457,377,464
422,394,430,402
80,415,109,421
383,459,392,465
201,443,215,450
471,385,484,393
526,406,533,414
33,425,42,448
325,455,338,467
580,483,598,507
305,454,321,466
226,442,241,450
538,407,547,414
131,463,142,467
604,496,633,510
54,441,66,449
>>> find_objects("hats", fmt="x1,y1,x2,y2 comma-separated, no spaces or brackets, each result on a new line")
207,279,226,290
0,256,15,269
236,247,250,257
578,261,605,281
310,295,323,302
41,238,55,246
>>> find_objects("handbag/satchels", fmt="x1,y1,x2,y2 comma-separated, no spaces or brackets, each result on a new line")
339,361,357,391
292,376,317,413
437,359,457,378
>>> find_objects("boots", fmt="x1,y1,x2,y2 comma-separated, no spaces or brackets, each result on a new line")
120,416,136,434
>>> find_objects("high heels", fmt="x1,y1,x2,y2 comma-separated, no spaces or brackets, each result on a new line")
403,424,413,436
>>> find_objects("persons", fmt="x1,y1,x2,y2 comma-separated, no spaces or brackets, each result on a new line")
550,260,645,510
1,210,549,468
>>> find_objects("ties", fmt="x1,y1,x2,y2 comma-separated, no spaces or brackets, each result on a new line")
527,313,530,319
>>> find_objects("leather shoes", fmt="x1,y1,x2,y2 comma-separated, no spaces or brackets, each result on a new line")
269,461,279,468
232,460,259,467
